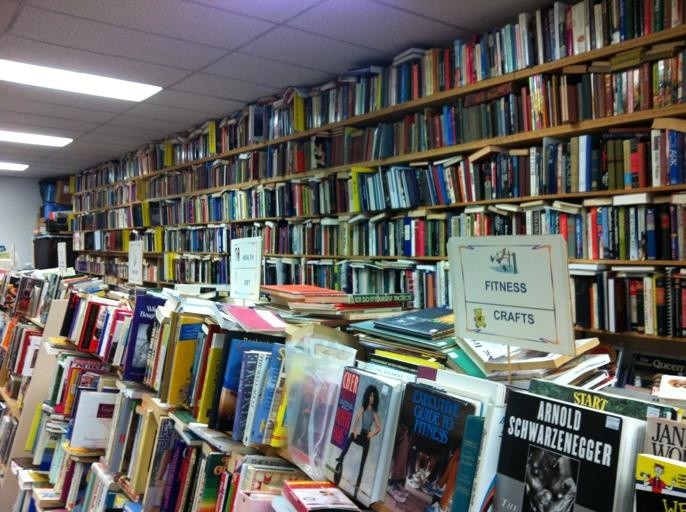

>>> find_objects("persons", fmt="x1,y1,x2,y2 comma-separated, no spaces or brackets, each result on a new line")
335,385,381,498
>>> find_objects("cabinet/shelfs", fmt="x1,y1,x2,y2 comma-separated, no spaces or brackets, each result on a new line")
0,0,686,512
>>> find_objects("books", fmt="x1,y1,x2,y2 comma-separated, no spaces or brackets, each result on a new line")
72,4,684,339
1,272,684,510
